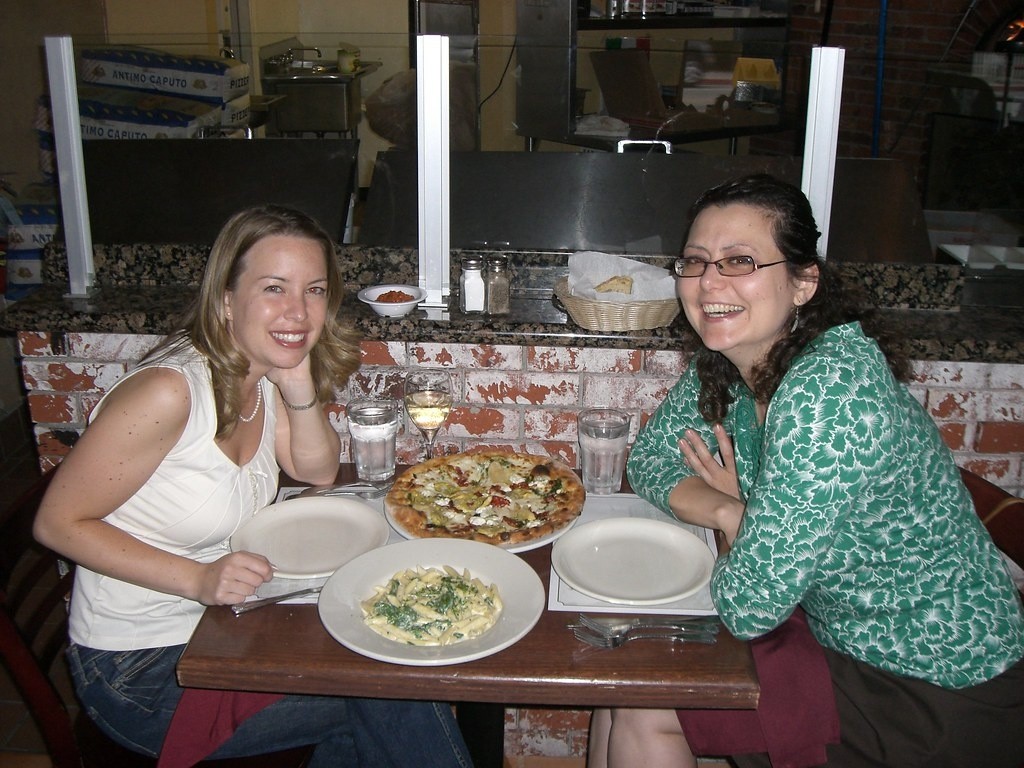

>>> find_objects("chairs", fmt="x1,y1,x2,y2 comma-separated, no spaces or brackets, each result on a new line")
961,466,1024,597
0,467,317,768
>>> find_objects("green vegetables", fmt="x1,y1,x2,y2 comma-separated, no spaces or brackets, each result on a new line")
370,576,495,647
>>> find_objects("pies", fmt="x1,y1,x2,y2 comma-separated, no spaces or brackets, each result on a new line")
594,276,633,294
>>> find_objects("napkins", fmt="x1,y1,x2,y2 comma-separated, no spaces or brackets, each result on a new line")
157,688,282,768
671,604,842,768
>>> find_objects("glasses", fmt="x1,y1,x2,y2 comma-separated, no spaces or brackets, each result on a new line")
675,256,790,278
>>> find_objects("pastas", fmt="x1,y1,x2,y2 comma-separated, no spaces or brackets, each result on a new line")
360,563,503,647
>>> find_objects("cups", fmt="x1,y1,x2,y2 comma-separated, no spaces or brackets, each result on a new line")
346,395,399,481
578,409,630,494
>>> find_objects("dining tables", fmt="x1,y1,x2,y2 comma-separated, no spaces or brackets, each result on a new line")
177,464,761,768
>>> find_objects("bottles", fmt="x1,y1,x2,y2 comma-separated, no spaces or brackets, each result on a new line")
486,258,510,314
460,254,487,314
606,0,714,18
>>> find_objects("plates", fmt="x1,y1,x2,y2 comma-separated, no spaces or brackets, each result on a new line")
384,461,585,553
317,538,545,667
230,495,390,578
750,102,778,113
551,518,715,606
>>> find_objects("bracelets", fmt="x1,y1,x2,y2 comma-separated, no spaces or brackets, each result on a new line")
283,395,317,409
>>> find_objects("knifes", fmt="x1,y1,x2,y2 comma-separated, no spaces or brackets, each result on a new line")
579,613,720,636
231,585,324,615
567,617,707,628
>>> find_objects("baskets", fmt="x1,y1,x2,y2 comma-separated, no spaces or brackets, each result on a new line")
553,278,682,333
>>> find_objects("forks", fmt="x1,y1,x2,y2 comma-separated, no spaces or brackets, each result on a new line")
574,627,718,649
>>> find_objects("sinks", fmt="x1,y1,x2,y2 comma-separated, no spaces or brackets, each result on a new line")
248,94,273,104
311,63,371,75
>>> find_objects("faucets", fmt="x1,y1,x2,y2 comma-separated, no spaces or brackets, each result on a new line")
286,46,322,58
218,46,235,60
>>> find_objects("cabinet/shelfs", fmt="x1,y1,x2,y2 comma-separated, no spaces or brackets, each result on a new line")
514,0,791,152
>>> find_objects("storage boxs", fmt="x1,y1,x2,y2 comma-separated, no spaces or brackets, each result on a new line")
0,46,251,312
920,209,1021,261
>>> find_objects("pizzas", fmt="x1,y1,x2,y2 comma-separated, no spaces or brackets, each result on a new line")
385,448,588,547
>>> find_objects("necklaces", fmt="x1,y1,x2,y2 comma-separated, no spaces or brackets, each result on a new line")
240,381,261,422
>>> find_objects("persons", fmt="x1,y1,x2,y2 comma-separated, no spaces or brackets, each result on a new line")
586,173,1024,767
33,203,477,768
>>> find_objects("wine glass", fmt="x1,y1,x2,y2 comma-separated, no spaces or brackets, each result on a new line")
402,369,453,459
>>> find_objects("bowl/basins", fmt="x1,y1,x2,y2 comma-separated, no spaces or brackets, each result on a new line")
358,284,427,317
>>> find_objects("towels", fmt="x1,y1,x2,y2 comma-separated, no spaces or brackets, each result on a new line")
575,113,629,137
